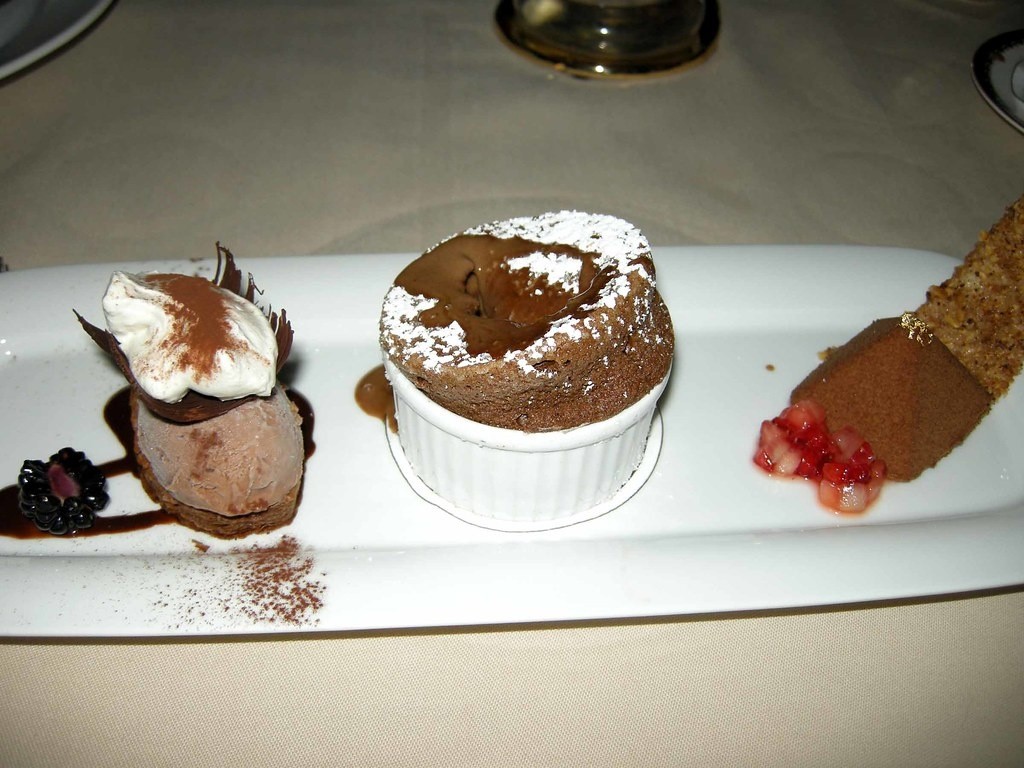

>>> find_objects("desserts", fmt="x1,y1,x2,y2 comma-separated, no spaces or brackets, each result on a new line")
752,195,1024,514
61,239,305,538
380,208,674,432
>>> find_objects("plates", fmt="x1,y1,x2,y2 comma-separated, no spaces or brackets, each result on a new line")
384,405,662,533
970,29,1024,133
0,247,1023,639
0,0,111,79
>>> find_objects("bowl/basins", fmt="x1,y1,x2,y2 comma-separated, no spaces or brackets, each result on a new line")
381,348,673,524
